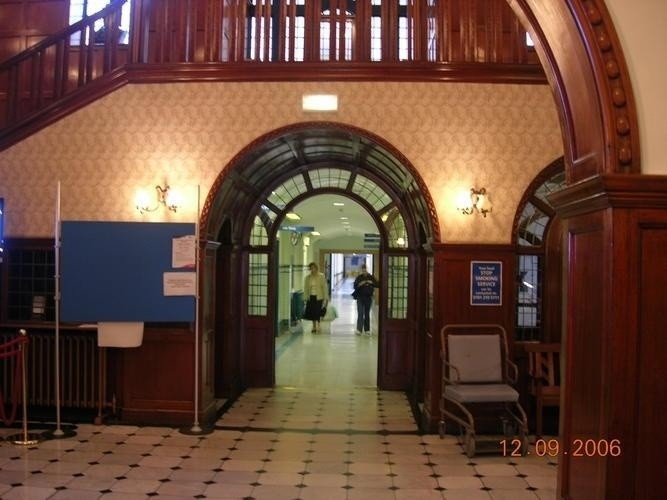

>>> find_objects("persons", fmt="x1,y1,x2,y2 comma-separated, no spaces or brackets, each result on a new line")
304,262,329,333
354,264,378,337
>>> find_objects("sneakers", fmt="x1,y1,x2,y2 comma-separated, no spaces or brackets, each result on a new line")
355,330,371,336
311,328,322,333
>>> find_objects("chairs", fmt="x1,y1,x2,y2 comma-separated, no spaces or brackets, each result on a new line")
523,343,561,443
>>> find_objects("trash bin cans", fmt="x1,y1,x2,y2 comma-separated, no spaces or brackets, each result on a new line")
293,291,304,315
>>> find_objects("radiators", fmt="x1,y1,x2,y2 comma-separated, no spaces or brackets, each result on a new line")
0,331,116,425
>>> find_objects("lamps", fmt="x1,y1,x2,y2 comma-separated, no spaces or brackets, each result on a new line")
453,185,492,220
132,183,181,216
